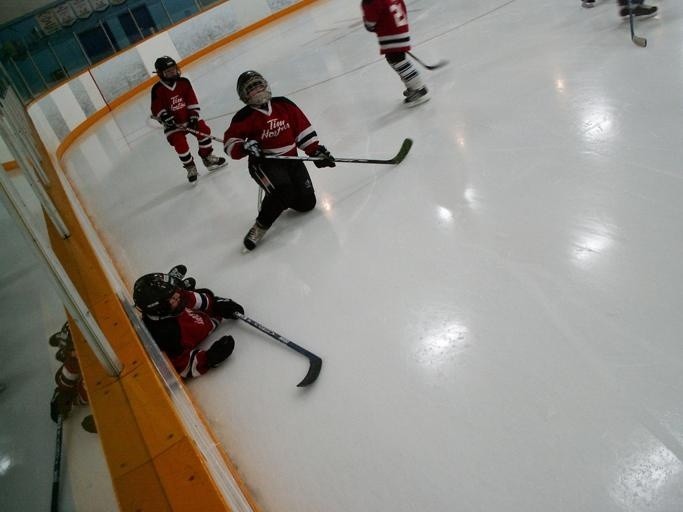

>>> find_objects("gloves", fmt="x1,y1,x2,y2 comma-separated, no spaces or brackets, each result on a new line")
51,388,79,422
204,335,234,369
160,110,176,129
241,139,265,163
213,296,244,319
309,145,335,167
188,116,200,134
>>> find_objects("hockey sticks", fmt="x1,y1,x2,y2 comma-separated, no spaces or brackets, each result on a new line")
628,1,647,48
234,311,322,386
407,51,448,70
265,138,413,165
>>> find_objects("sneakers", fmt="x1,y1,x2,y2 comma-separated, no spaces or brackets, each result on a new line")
168,265,186,280
402,88,428,102
619,3,657,16
244,216,271,249
49,332,61,347
198,155,226,168
56,348,67,361
183,165,197,183
182,278,195,291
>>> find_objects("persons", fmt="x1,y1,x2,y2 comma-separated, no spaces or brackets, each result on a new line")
134,265,244,381
224,70,335,250
151,55,226,181
581,0,657,17
361,0,428,104
49,322,95,433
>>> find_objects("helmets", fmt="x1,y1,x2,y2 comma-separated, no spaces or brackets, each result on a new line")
156,56,181,83
133,273,185,317
237,70,272,107
